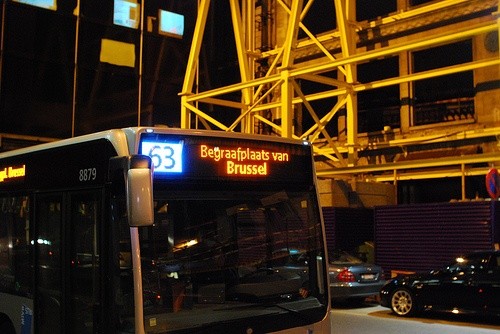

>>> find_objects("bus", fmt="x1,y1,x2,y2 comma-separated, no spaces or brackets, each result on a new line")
0,126,330,334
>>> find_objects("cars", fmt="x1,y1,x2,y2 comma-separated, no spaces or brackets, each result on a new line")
328,248,385,306
379,248,500,318
